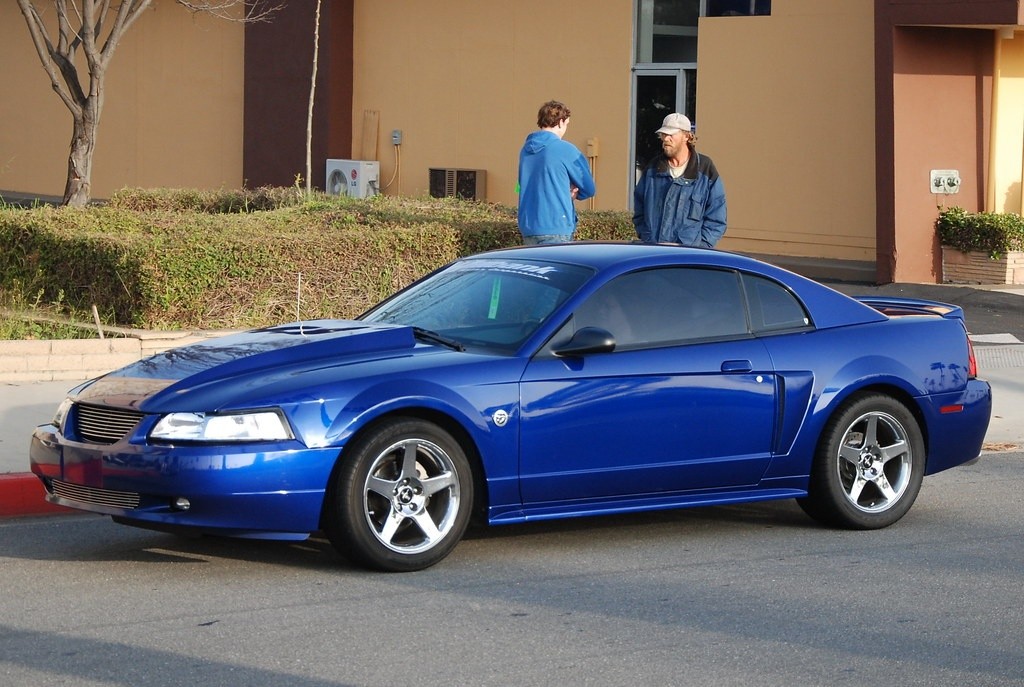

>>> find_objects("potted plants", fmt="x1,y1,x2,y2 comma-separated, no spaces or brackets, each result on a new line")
937,206,1024,285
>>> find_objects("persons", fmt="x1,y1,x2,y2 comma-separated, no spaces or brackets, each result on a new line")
595,286,641,343
633,113,728,247
517,100,595,244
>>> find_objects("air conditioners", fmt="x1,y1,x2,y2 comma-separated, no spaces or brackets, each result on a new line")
326,158,380,199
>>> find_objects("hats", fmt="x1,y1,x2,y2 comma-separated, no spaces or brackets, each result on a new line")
654,113,691,135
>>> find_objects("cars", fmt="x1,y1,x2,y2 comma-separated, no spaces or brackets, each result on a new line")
27,241,993,573
